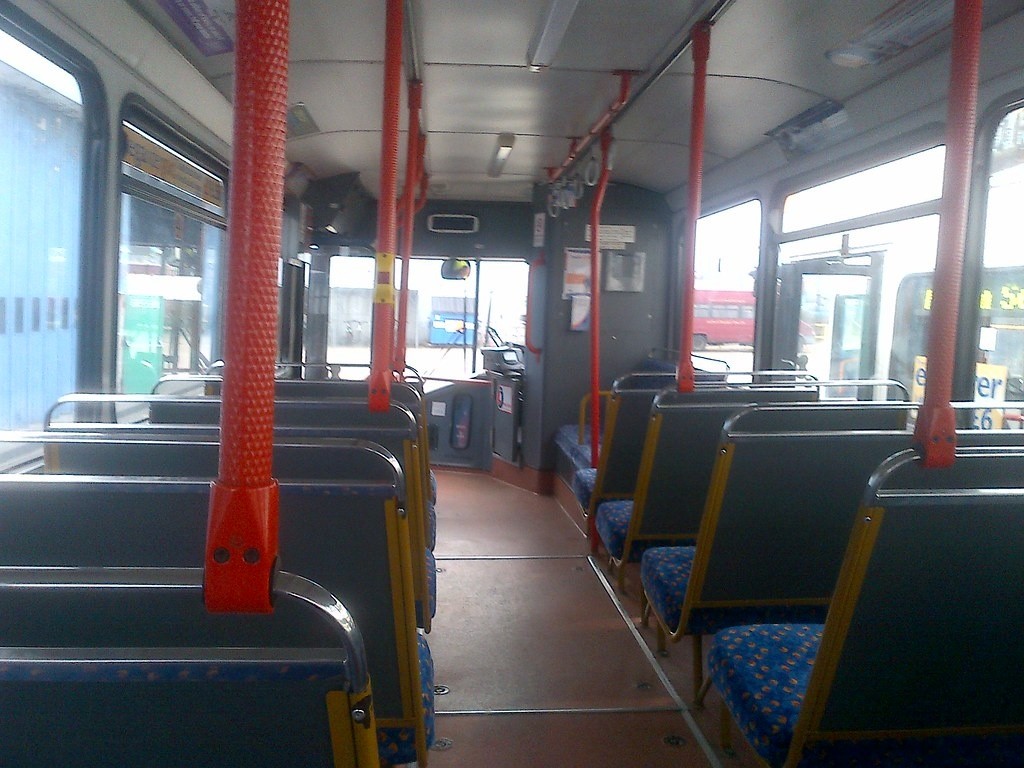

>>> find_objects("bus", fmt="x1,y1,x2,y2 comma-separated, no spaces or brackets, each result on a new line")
0,59,306,434
886,264,1023,434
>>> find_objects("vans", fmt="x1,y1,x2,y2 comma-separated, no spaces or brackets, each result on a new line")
693,289,816,353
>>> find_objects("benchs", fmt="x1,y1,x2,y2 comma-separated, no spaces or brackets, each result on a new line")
0,360,441,768
558,346,1024,766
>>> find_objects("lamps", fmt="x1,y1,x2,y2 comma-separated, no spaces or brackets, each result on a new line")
525,0,579,74
487,132,514,177
